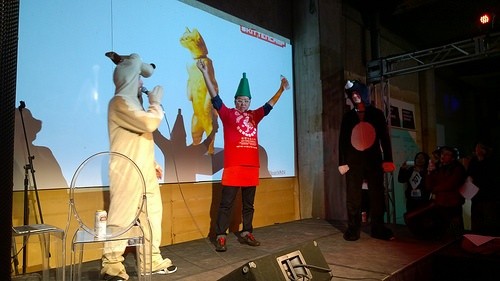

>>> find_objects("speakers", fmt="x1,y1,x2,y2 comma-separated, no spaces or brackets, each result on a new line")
405,203,444,238
217,240,333,281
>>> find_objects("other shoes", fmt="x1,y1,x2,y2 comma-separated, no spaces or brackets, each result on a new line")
104,274,125,281
239,231,259,246
216,234,228,251
139,265,178,275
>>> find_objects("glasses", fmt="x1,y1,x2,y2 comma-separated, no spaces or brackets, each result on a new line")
235,99,251,103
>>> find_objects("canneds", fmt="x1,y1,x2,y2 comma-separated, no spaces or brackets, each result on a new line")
94,210,108,228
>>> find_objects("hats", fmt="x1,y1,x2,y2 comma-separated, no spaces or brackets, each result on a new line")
234,71,252,98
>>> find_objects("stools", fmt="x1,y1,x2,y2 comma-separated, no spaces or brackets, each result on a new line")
12,222,64,281
70,226,146,281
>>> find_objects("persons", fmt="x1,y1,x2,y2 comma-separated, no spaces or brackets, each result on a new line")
339,79,398,240
101,51,177,281
196,60,288,252
398,152,432,212
428,144,500,236
425,148,464,235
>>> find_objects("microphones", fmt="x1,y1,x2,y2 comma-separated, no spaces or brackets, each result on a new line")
142,87,149,95
